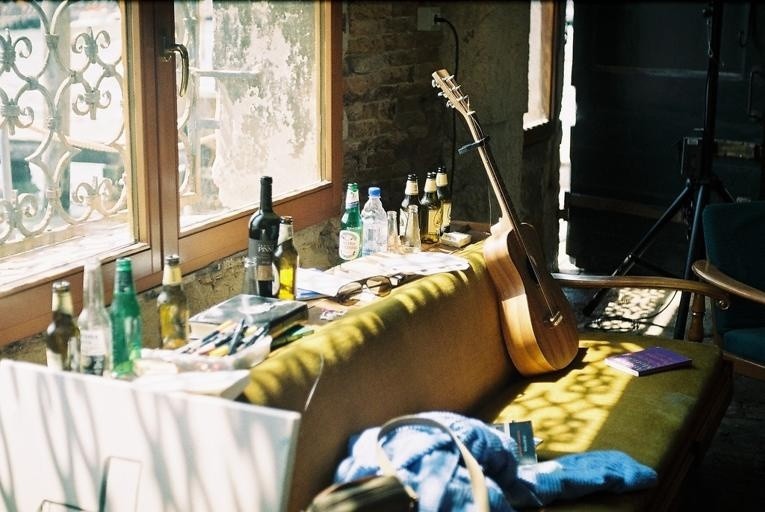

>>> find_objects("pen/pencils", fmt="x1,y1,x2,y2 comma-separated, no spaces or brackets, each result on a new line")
181,318,270,356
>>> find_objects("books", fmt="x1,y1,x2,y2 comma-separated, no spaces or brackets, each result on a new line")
604,346,693,378
188,293,314,348
487,421,538,466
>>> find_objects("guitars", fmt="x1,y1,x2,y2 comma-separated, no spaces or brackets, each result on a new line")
431,66,580,375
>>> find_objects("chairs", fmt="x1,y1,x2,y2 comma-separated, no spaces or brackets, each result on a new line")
691,200,765,380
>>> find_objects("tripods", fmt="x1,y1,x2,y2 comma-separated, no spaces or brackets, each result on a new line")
583,1,737,339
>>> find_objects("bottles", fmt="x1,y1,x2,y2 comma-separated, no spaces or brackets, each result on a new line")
78,259,111,376
248,177,280,299
336,165,452,266
271,215,297,301
110,258,142,376
47,282,80,373
242,257,260,296
157,255,188,351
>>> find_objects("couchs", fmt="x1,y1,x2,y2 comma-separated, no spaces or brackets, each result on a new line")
243,236,734,512
